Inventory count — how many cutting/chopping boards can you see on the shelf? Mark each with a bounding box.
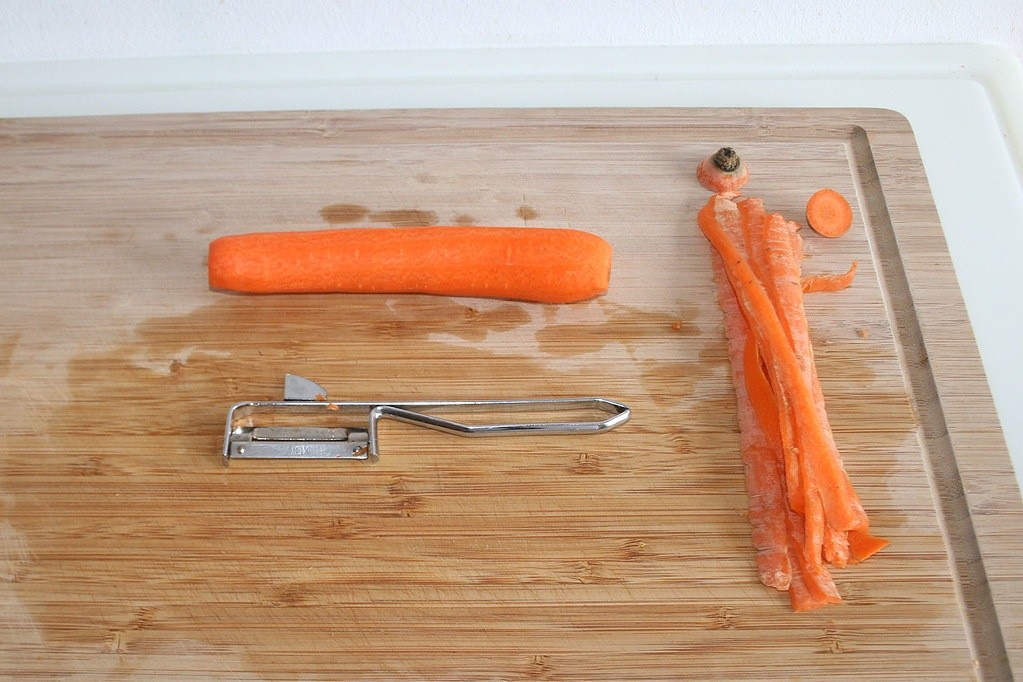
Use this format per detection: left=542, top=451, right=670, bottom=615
left=3, top=107, right=1023, bottom=681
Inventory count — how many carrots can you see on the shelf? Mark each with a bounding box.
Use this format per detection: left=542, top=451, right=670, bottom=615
left=209, top=225, right=611, bottom=303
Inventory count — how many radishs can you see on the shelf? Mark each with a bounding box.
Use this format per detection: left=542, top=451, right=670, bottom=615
left=804, top=188, right=852, bottom=237
